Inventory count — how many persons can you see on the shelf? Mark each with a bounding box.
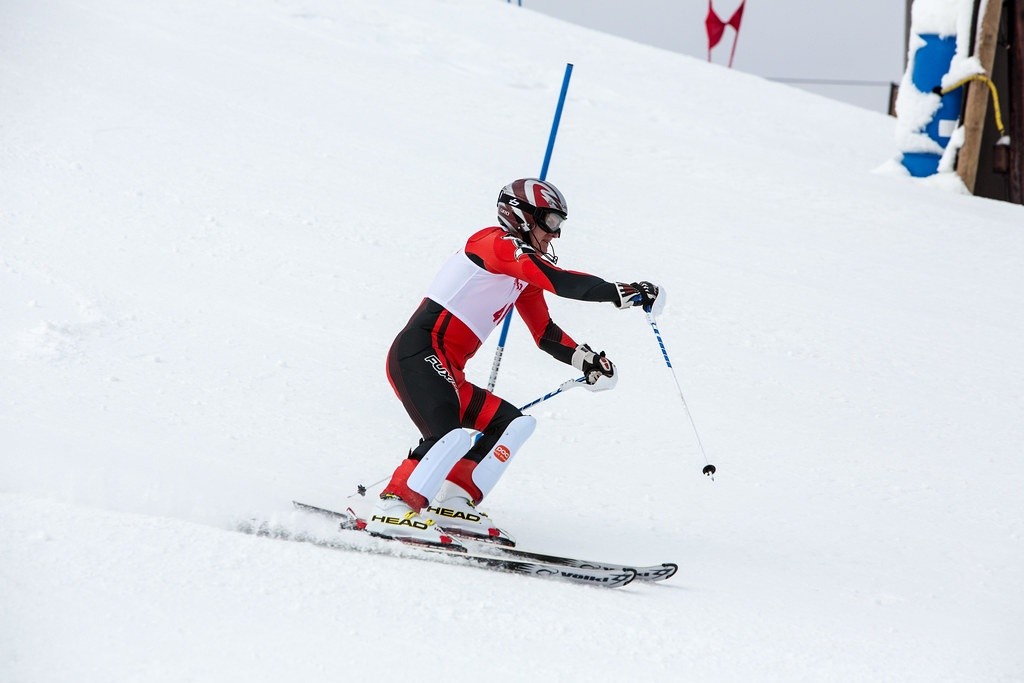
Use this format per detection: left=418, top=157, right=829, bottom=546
left=365, top=176, right=668, bottom=550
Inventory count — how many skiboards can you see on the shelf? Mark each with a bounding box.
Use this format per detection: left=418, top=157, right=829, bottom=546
left=240, top=499, right=679, bottom=589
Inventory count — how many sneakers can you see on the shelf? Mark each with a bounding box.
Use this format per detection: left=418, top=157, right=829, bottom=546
left=423, top=496, right=516, bottom=547
left=364, top=497, right=463, bottom=547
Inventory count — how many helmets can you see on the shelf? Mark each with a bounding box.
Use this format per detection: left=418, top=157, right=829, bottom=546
left=496, top=178, right=568, bottom=233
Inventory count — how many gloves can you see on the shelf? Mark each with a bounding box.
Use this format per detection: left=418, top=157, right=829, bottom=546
left=613, top=280, right=658, bottom=313
left=572, top=341, right=614, bottom=385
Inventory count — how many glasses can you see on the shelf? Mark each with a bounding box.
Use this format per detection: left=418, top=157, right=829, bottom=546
left=500, top=194, right=567, bottom=238
